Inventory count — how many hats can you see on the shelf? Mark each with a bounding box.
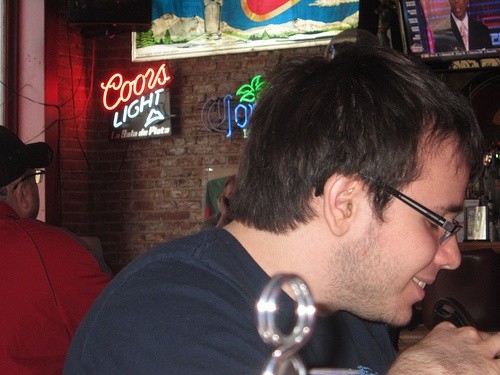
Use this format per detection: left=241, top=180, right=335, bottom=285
left=0, top=125, right=54, bottom=188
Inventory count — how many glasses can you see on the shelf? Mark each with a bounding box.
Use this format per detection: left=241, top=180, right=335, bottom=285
left=14, top=171, right=45, bottom=190
left=314, top=168, right=462, bottom=246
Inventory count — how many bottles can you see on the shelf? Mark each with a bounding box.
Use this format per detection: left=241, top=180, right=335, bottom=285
left=449, top=150, right=500, bottom=244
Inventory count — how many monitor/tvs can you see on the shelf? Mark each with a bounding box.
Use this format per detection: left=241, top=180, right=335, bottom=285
left=396, top=0, right=500, bottom=71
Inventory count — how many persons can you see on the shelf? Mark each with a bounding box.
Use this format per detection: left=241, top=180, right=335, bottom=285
left=432, top=0, right=493, bottom=53
left=61, top=28, right=500, bottom=375
left=0, top=126, right=120, bottom=375
left=198, top=175, right=235, bottom=232
